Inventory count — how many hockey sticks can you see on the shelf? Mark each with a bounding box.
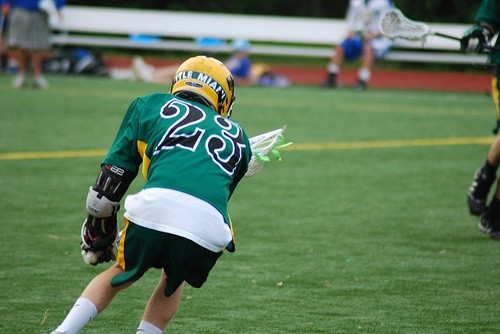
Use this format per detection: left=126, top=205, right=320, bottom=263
left=382, top=9, right=500, bottom=51
left=115, top=125, right=293, bottom=244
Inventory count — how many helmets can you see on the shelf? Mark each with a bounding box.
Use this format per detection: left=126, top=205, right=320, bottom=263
left=171, top=56, right=235, bottom=119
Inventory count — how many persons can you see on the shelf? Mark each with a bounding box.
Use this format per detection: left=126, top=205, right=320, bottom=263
left=0, top=0, right=65, bottom=89
left=49, top=55, right=253, bottom=334
left=131, top=38, right=255, bottom=86
left=460, top=0, right=500, bottom=239
left=321, top=0, right=398, bottom=90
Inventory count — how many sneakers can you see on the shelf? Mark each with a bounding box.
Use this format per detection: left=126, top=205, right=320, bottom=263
left=479, top=179, right=500, bottom=231
left=316, top=73, right=337, bottom=87
left=9, top=72, right=27, bottom=90
left=345, top=79, right=366, bottom=91
left=30, top=75, right=50, bottom=92
left=468, top=166, right=497, bottom=217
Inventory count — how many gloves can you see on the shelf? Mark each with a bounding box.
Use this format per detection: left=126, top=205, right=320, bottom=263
left=81, top=220, right=120, bottom=266
left=460, top=25, right=495, bottom=55
